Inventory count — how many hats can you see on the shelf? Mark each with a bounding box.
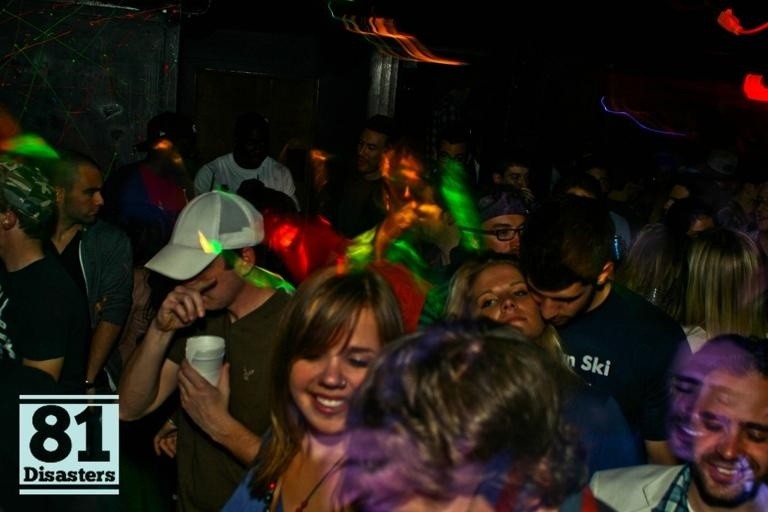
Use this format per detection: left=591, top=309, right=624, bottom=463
left=136, top=110, right=198, bottom=154
left=706, top=150, right=738, bottom=174
left=1, top=158, right=55, bottom=224
left=144, top=190, right=264, bottom=279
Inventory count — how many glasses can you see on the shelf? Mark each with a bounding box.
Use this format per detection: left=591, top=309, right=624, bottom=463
left=482, top=228, right=526, bottom=241
left=600, top=178, right=609, bottom=185
left=753, top=199, right=768, bottom=206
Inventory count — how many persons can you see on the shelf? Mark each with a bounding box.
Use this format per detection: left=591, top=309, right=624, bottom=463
left=220, top=269, right=404, bottom=512
left=446, top=257, right=641, bottom=499
left=345, top=113, right=768, bottom=339
left=333, top=321, right=586, bottom=511
left=1, top=350, right=74, bottom=512
left=52, top=155, right=133, bottom=394
left=558, top=347, right=768, bottom=512
left=116, top=190, right=298, bottom=512
left=0, top=154, right=81, bottom=387
left=518, top=194, right=683, bottom=464
left=127, top=113, right=330, bottom=187
